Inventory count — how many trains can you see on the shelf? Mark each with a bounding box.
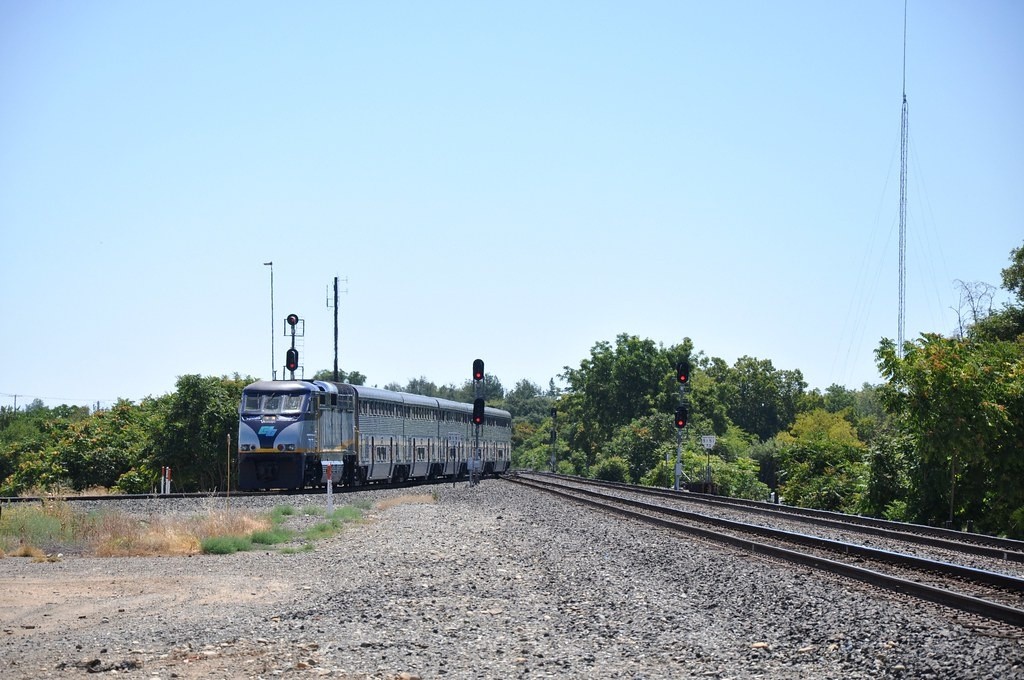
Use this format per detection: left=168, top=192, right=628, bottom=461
left=236, top=378, right=512, bottom=493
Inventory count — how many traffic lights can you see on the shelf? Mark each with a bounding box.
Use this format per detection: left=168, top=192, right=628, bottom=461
left=286, top=348, right=299, bottom=371
left=472, top=398, right=485, bottom=425
left=675, top=406, right=687, bottom=429
left=472, top=359, right=484, bottom=380
left=287, top=314, right=299, bottom=325
left=676, top=362, right=690, bottom=384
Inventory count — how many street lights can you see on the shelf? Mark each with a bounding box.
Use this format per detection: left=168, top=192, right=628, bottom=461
left=264, top=261, right=275, bottom=380
